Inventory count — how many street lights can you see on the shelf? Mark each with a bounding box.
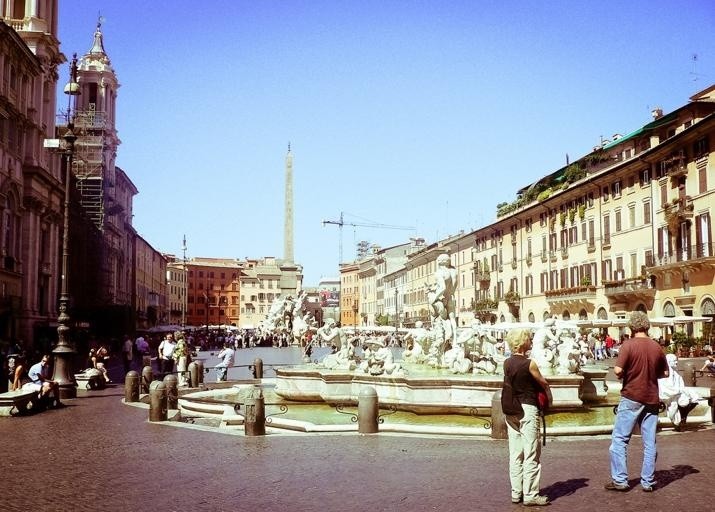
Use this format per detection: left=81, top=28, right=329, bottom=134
left=181, top=235, right=187, bottom=338
left=46, top=52, right=80, bottom=400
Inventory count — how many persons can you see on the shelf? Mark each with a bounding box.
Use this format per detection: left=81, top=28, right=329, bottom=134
left=699, top=353, right=715, bottom=376
left=654, top=336, right=671, bottom=347
left=658, top=353, right=690, bottom=427
left=578, top=334, right=630, bottom=366
left=503, top=328, right=553, bottom=506
left=318, top=254, right=583, bottom=375
left=605, top=311, right=669, bottom=491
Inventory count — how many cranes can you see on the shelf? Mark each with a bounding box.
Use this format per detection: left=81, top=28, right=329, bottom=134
left=322, top=212, right=416, bottom=280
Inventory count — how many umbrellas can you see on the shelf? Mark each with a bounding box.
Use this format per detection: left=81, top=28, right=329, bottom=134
left=577, top=316, right=713, bottom=337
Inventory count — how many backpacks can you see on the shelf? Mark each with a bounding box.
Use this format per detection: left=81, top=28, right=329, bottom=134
left=501, top=358, right=530, bottom=416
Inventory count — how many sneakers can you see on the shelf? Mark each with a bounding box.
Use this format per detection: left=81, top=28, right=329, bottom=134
left=604, top=480, right=628, bottom=491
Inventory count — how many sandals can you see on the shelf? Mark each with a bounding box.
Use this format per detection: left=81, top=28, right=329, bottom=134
left=510, top=492, right=550, bottom=506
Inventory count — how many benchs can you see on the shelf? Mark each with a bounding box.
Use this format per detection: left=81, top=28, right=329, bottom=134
left=74, top=371, right=106, bottom=390
left=1, top=383, right=42, bottom=417
